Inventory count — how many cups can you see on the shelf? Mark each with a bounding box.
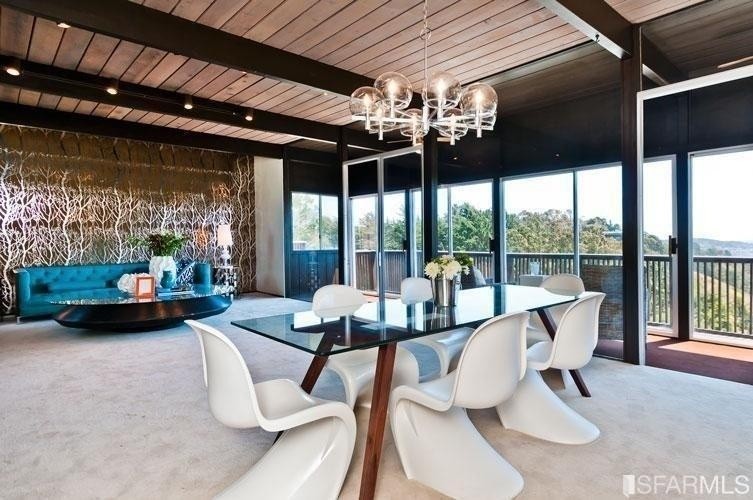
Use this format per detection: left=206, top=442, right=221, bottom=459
left=530, top=262, right=539, bottom=276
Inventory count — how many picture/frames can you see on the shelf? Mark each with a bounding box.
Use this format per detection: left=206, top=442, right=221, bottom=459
left=135, top=276, right=155, bottom=296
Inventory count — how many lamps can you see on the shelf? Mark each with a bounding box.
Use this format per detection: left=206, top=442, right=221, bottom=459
left=216, top=224, right=233, bottom=266
left=346, top=0, right=499, bottom=148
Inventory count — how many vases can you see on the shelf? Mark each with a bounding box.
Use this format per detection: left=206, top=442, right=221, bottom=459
left=116, top=272, right=149, bottom=293
left=434, top=275, right=461, bottom=307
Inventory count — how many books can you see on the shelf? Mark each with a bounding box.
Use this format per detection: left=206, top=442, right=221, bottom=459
left=157, top=286, right=193, bottom=296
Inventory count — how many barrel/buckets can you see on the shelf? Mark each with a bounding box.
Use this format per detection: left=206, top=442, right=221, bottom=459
left=193, top=263, right=211, bottom=285
left=433, top=276, right=459, bottom=307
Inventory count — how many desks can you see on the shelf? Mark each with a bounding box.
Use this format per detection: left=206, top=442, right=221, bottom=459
left=229, top=282, right=593, bottom=500
left=44, top=284, right=234, bottom=333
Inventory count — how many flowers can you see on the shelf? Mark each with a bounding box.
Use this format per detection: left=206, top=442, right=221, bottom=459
left=423, top=252, right=471, bottom=279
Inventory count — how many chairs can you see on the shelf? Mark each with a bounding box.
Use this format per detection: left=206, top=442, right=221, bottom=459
left=494, top=290, right=606, bottom=447
left=388, top=308, right=533, bottom=499
left=579, top=263, right=649, bottom=341
left=399, top=276, right=477, bottom=383
left=311, top=284, right=420, bottom=410
left=183, top=319, right=357, bottom=500
left=526, top=274, right=584, bottom=391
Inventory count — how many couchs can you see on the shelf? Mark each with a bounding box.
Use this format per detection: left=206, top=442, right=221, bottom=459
left=11, top=260, right=210, bottom=326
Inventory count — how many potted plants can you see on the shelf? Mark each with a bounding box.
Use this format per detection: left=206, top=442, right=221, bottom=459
left=126, top=229, right=190, bottom=287
